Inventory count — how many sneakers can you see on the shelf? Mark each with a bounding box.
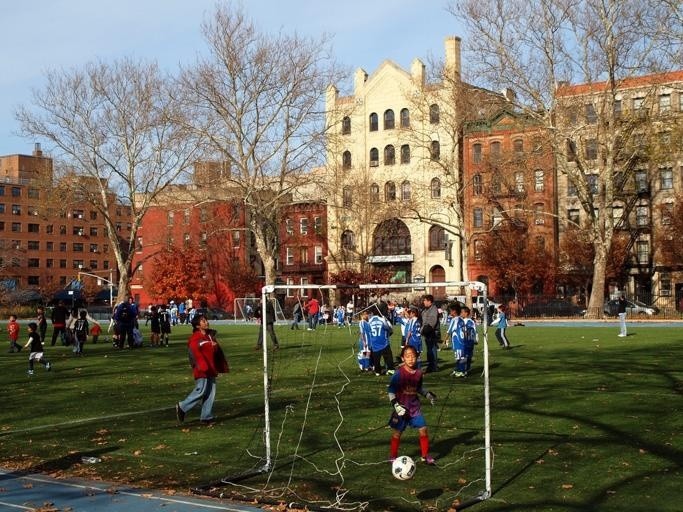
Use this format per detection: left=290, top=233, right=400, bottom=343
left=449, top=369, right=472, bottom=378
left=176, top=402, right=185, bottom=424
left=375, top=370, right=395, bottom=377
left=45, top=362, right=51, bottom=371
left=420, top=455, right=438, bottom=466
left=358, top=363, right=372, bottom=372
left=199, top=419, right=217, bottom=426
left=8, top=346, right=22, bottom=353
left=26, top=369, right=34, bottom=375
left=291, top=327, right=315, bottom=331
left=256, top=345, right=279, bottom=352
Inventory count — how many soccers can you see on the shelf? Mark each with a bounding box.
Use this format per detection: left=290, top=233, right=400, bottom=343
left=392, top=455, right=417, bottom=481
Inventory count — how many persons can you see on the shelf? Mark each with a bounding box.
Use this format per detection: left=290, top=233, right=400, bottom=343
left=356, top=293, right=511, bottom=380
left=252, top=296, right=280, bottom=351
left=242, top=291, right=356, bottom=333
left=174, top=314, right=231, bottom=427
left=387, top=346, right=438, bottom=468
left=20, top=322, right=52, bottom=376
left=5, top=295, right=197, bottom=357
left=614, top=293, right=631, bottom=341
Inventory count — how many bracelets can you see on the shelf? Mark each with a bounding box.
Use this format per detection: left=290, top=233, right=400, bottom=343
left=390, top=398, right=397, bottom=408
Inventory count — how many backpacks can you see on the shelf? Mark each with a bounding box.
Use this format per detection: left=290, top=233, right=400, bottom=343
left=119, top=303, right=131, bottom=322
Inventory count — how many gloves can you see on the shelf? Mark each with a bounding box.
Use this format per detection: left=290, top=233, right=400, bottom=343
left=394, top=401, right=409, bottom=417
left=424, top=391, right=438, bottom=406
